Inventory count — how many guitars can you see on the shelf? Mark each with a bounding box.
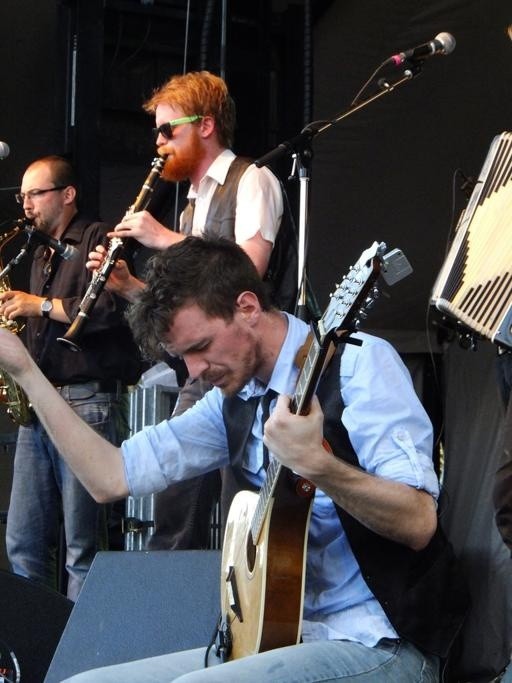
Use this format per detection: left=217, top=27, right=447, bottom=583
left=217, top=242, right=413, bottom=664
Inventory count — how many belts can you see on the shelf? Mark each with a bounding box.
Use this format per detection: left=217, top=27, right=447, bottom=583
left=56, top=379, right=122, bottom=402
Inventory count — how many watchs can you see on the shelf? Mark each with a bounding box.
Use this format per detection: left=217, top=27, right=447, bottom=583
left=40, top=296, right=53, bottom=319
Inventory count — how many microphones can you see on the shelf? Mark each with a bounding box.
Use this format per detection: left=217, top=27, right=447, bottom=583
left=0, top=140, right=10, bottom=161
left=25, top=222, right=80, bottom=263
left=389, top=31, right=456, bottom=67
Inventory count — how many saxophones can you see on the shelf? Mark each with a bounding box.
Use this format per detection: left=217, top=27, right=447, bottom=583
left=0, top=217, right=38, bottom=426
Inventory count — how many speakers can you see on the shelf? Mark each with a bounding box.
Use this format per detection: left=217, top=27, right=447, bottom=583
left=43, top=549, right=222, bottom=683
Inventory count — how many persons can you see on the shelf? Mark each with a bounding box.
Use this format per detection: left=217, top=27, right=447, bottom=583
left=85, top=69, right=295, bottom=554
left=0, top=234, right=472, bottom=683
left=1, top=155, right=132, bottom=605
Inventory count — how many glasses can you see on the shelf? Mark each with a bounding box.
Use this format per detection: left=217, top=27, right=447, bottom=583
left=150, top=114, right=217, bottom=141
left=15, top=185, right=67, bottom=204
